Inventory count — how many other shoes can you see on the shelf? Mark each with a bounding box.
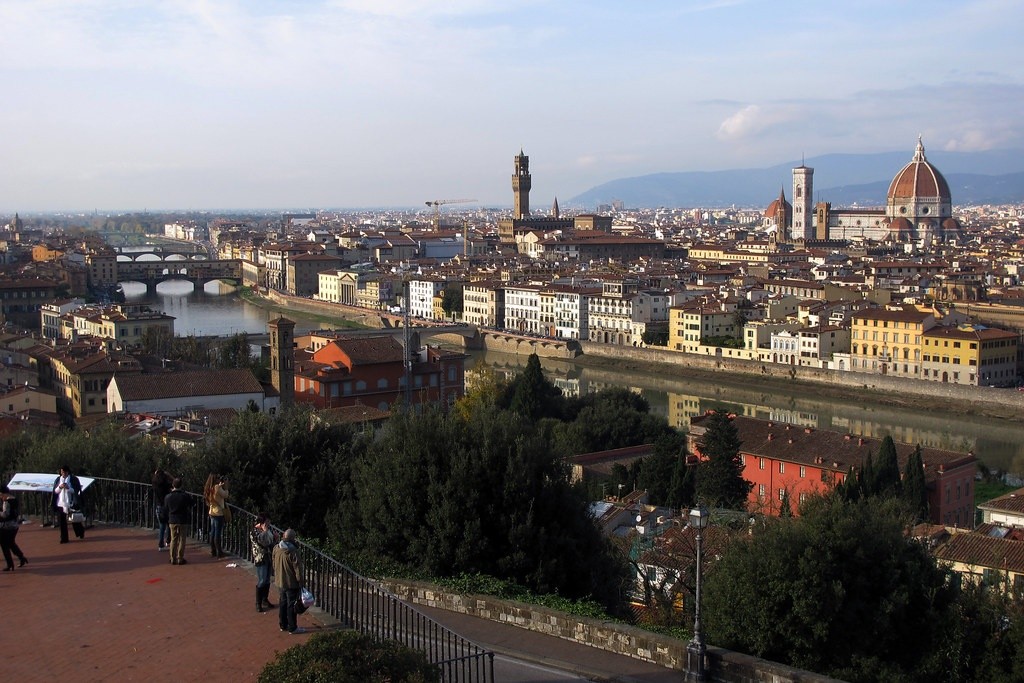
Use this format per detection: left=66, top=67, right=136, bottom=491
left=289, top=627, right=305, bottom=634
left=170, top=561, right=177, bottom=565
left=280, top=625, right=290, bottom=632
left=80, top=530, right=85, bottom=539
left=178, top=560, right=188, bottom=565
left=58, top=539, right=70, bottom=544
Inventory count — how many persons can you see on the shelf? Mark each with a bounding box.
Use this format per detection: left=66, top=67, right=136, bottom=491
left=203, top=472, right=232, bottom=558
left=0, top=486, right=28, bottom=571
left=53, top=465, right=85, bottom=544
left=164, top=478, right=195, bottom=565
left=272, top=529, right=307, bottom=634
left=152, top=468, right=172, bottom=551
left=250, top=513, right=280, bottom=612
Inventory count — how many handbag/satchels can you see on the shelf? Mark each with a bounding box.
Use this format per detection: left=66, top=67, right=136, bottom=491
left=267, top=542, right=277, bottom=565
left=223, top=507, right=231, bottom=523
left=293, top=587, right=314, bottom=614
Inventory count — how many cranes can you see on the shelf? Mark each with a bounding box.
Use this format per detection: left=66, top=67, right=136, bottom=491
left=425, top=199, right=478, bottom=233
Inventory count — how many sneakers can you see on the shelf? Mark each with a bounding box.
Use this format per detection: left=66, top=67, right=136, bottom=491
left=165, top=543, right=170, bottom=547
left=159, top=547, right=167, bottom=551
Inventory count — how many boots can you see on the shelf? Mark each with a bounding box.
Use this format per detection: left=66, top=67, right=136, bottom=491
left=210, top=539, right=217, bottom=558
left=256, top=585, right=266, bottom=613
left=262, top=584, right=274, bottom=608
left=214, top=539, right=226, bottom=557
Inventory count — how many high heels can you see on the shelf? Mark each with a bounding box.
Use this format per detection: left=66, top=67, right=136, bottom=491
left=2, top=564, right=15, bottom=572
left=17, top=557, right=29, bottom=568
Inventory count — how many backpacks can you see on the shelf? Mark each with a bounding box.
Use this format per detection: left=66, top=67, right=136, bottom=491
left=66, top=490, right=86, bottom=523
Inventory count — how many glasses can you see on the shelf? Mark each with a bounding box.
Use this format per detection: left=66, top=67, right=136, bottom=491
left=217, top=477, right=221, bottom=480
